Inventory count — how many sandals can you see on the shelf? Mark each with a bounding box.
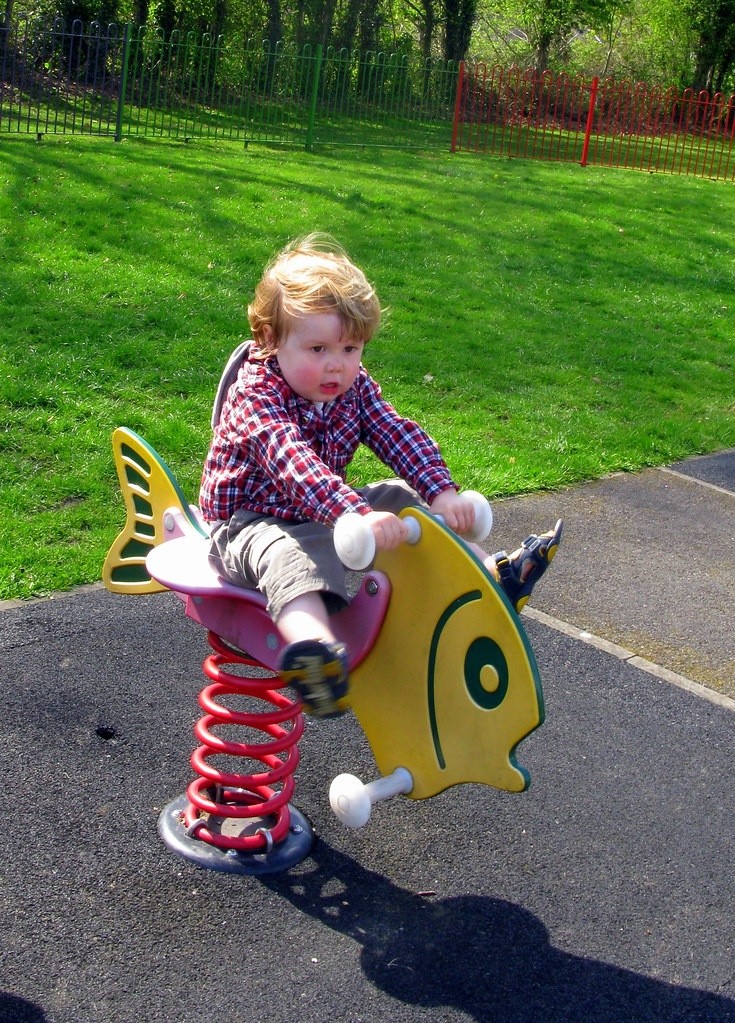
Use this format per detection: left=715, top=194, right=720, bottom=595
left=491, top=517, right=563, bottom=616
left=281, top=639, right=351, bottom=720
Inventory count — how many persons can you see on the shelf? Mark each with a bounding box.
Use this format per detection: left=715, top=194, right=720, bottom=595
left=195, top=230, right=565, bottom=721
